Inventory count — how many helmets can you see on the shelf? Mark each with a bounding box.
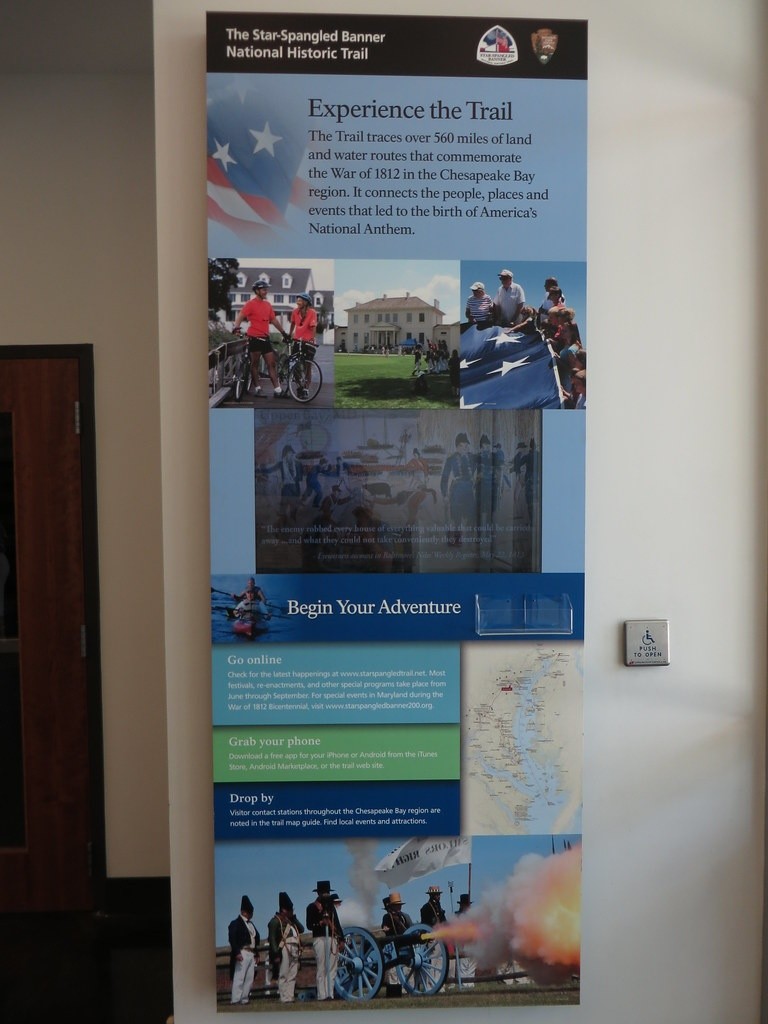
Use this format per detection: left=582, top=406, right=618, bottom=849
left=296, top=293, right=313, bottom=305
left=252, top=280, right=271, bottom=289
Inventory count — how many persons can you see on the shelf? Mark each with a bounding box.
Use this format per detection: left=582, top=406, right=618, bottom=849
left=232, top=577, right=271, bottom=623
left=465, top=281, right=493, bottom=323
left=232, top=280, right=318, bottom=399
left=381, top=337, right=423, bottom=376
left=380, top=886, right=535, bottom=998
left=424, top=338, right=460, bottom=398
left=493, top=269, right=586, bottom=409
left=227, top=880, right=346, bottom=1005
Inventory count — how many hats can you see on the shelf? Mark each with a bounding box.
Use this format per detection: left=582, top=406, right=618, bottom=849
left=381, top=897, right=391, bottom=909
left=240, top=895, right=254, bottom=911
left=470, top=282, right=484, bottom=291
left=425, top=886, right=443, bottom=894
left=457, top=894, right=472, bottom=904
left=279, top=892, right=293, bottom=907
left=389, top=894, right=406, bottom=905
left=245, top=586, right=255, bottom=593
left=497, top=269, right=513, bottom=278
left=330, top=894, right=342, bottom=903
left=312, top=881, right=335, bottom=892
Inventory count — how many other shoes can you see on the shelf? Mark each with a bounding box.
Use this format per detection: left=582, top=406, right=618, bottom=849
left=275, top=389, right=289, bottom=399
left=255, top=388, right=266, bottom=398
left=303, top=389, right=309, bottom=399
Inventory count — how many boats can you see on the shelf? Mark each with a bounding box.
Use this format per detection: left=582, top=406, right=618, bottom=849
left=233, top=619, right=270, bottom=639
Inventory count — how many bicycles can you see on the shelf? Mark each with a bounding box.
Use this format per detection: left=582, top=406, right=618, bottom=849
left=233, top=337, right=322, bottom=404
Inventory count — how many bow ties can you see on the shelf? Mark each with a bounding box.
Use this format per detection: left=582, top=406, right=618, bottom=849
left=245, top=920, right=250, bottom=923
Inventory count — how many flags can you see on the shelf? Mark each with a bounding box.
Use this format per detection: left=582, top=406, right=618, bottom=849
left=374, top=835, right=471, bottom=890
